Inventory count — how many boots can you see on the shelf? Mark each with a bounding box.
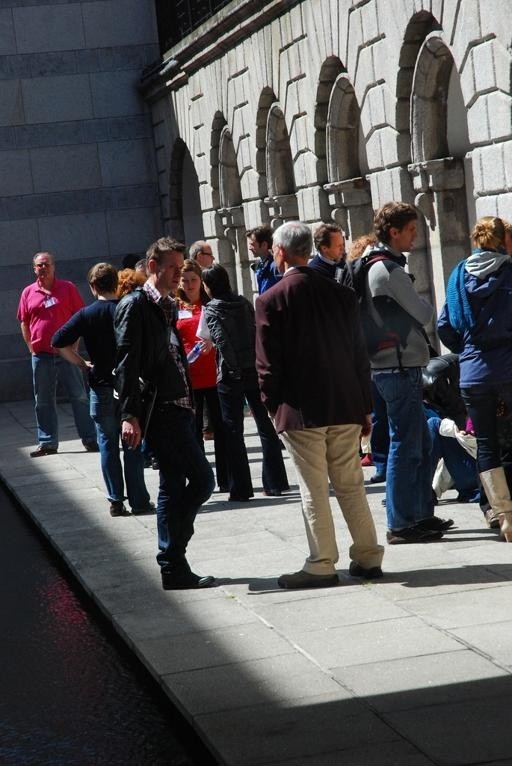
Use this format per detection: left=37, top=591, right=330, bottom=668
left=482, top=462, right=512, bottom=545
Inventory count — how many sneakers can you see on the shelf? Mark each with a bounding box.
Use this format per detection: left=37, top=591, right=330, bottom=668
left=85, top=442, right=99, bottom=453
left=130, top=498, right=156, bottom=515
left=30, top=444, right=47, bottom=457
left=108, top=501, right=127, bottom=518
left=347, top=561, right=384, bottom=580
left=276, top=571, right=340, bottom=588
left=216, top=481, right=285, bottom=502
left=161, top=568, right=215, bottom=592
left=385, top=513, right=456, bottom=545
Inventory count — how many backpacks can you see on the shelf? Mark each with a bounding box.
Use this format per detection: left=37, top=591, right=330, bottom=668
left=336, top=250, right=405, bottom=356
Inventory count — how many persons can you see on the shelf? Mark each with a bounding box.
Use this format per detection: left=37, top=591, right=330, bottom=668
left=16, top=200, right=511, bottom=590
